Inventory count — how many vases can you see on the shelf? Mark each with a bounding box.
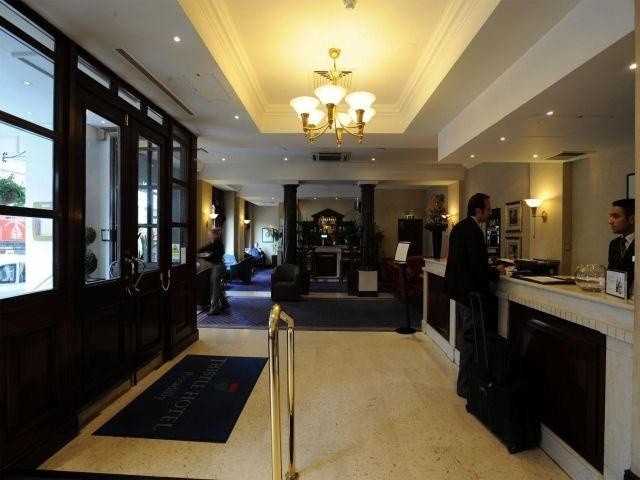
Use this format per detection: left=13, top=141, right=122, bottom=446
left=427, top=193, right=448, bottom=224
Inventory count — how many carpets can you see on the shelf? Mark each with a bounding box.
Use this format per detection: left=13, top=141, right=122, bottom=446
left=620, top=238, right=626, bottom=256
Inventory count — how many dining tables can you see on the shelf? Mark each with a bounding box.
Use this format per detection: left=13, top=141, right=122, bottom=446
left=296, top=219, right=313, bottom=295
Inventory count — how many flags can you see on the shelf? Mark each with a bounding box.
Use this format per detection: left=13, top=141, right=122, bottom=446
left=424, top=224, right=448, bottom=259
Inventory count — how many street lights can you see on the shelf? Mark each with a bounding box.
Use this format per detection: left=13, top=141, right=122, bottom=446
left=478, top=330, right=520, bottom=386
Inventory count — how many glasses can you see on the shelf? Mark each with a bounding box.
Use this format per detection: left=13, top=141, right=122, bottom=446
left=290, top=48, right=376, bottom=148
left=523, top=198, right=547, bottom=223
left=206, top=213, right=219, bottom=226
left=242, top=220, right=251, bottom=224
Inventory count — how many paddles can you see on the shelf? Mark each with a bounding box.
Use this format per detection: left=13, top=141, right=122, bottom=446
left=91, top=354, right=268, bottom=443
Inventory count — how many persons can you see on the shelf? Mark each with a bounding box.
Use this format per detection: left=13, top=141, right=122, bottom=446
left=441, top=191, right=508, bottom=399
left=197, top=227, right=231, bottom=316
left=603, top=196, right=636, bottom=299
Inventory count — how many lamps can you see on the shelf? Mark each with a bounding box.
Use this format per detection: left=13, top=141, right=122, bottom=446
left=271, top=264, right=301, bottom=301
left=230, top=257, right=255, bottom=284
left=223, top=253, right=238, bottom=284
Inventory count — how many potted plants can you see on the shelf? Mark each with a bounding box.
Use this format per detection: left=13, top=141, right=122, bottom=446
left=262, top=227, right=274, bottom=244
left=605, top=269, right=628, bottom=301
left=505, top=235, right=522, bottom=259
left=505, top=200, right=522, bottom=233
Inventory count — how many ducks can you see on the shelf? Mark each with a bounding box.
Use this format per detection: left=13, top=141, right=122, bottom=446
left=466, top=377, right=542, bottom=454
